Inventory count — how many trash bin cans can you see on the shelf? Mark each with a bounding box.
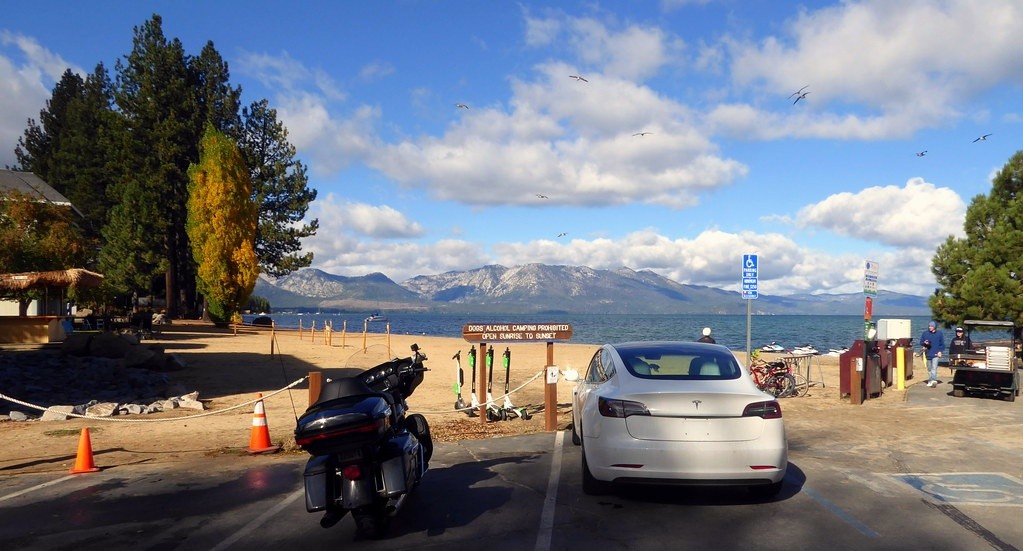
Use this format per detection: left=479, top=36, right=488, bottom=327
left=840, top=339, right=881, bottom=398
left=876, top=338, right=893, bottom=388
left=890, top=337, right=914, bottom=380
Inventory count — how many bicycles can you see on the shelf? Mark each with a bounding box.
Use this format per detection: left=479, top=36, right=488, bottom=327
left=744, top=347, right=811, bottom=400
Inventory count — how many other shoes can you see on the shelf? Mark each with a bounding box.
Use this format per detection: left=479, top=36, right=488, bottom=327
left=926, top=381, right=937, bottom=388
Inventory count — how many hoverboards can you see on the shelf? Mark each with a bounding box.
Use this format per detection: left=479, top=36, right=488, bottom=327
left=485, top=345, right=507, bottom=415
left=500, top=344, right=530, bottom=421
left=450, top=350, right=473, bottom=417
left=467, top=346, right=493, bottom=421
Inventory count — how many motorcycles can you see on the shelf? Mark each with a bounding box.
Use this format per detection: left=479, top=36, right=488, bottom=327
left=291, top=345, right=436, bottom=538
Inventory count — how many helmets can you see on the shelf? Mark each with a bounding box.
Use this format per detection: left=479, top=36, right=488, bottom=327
left=703, top=327, right=711, bottom=336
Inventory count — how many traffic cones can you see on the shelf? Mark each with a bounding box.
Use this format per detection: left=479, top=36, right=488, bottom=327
left=244, top=391, right=283, bottom=455
left=67, top=426, right=102, bottom=476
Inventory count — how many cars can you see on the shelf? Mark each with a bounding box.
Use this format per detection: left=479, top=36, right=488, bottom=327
left=948, top=319, right=1021, bottom=402
left=562, top=338, right=791, bottom=498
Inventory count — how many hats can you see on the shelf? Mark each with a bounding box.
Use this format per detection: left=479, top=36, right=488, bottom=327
left=928, top=322, right=935, bottom=328
left=954, top=328, right=964, bottom=332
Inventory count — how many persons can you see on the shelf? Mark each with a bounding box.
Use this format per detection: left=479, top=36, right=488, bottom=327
left=949, top=326, right=976, bottom=355
left=697, top=327, right=716, bottom=343
left=919, top=321, right=945, bottom=387
